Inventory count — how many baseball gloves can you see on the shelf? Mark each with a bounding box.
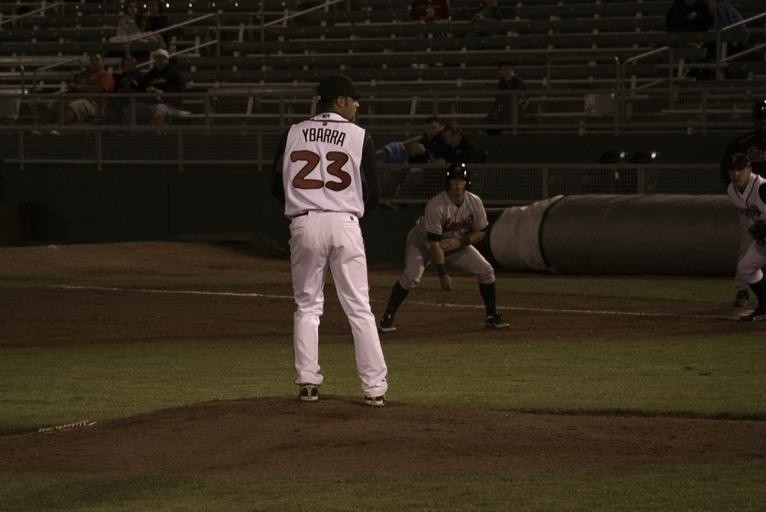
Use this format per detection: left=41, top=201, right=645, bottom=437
left=748, top=220, right=765, bottom=246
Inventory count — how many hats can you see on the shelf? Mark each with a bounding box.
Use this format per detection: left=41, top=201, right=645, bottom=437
left=316, top=73, right=370, bottom=101
left=727, top=153, right=751, bottom=171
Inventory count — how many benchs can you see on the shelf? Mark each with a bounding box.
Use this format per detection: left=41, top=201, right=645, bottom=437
left=0, top=0, right=764, bottom=168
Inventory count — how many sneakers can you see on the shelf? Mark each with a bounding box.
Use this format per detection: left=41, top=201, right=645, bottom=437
left=740, top=308, right=766, bottom=323
left=485, top=313, right=510, bottom=330
left=378, top=318, right=396, bottom=333
left=299, top=383, right=319, bottom=403
left=734, top=290, right=751, bottom=306
left=364, top=393, right=386, bottom=407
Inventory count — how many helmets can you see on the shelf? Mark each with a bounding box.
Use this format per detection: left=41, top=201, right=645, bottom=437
left=445, top=162, right=472, bottom=193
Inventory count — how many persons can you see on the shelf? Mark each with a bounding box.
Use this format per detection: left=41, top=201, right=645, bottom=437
left=410, top=0, right=457, bottom=43
left=62, top=1, right=185, bottom=125
left=410, top=115, right=464, bottom=165
left=378, top=159, right=510, bottom=335
left=484, top=53, right=533, bottom=126
left=723, top=148, right=766, bottom=326
left=279, top=74, right=391, bottom=409
left=470, top=0, right=503, bottom=41
